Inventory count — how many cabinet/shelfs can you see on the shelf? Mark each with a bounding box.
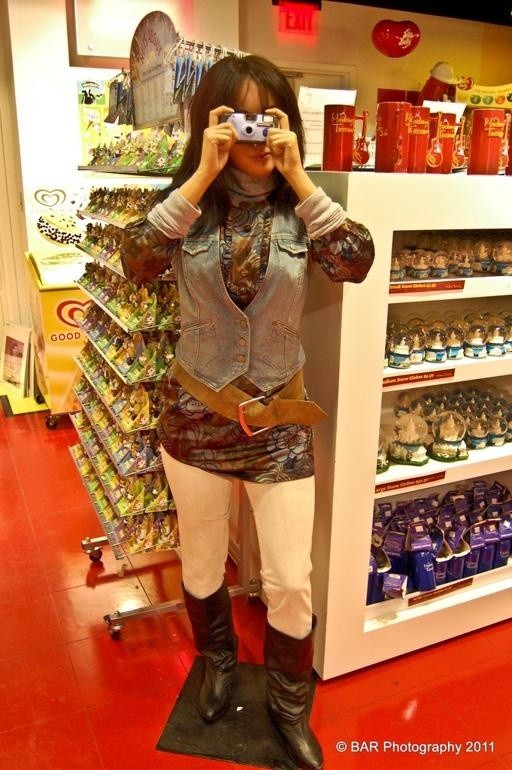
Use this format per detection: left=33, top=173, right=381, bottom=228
left=68, top=165, right=180, bottom=560
left=296, top=170, right=512, bottom=681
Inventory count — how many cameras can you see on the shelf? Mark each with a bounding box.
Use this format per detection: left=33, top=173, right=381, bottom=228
left=219, top=111, right=275, bottom=144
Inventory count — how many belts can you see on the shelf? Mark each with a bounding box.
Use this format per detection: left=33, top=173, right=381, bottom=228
left=170, top=356, right=329, bottom=443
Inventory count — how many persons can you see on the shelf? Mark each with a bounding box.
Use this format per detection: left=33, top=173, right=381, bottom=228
left=117, top=52, right=376, bottom=770
left=376, top=238, right=512, bottom=479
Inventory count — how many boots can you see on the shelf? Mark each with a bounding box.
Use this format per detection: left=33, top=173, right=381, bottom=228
left=179, top=573, right=241, bottom=725
left=261, top=613, right=325, bottom=769
